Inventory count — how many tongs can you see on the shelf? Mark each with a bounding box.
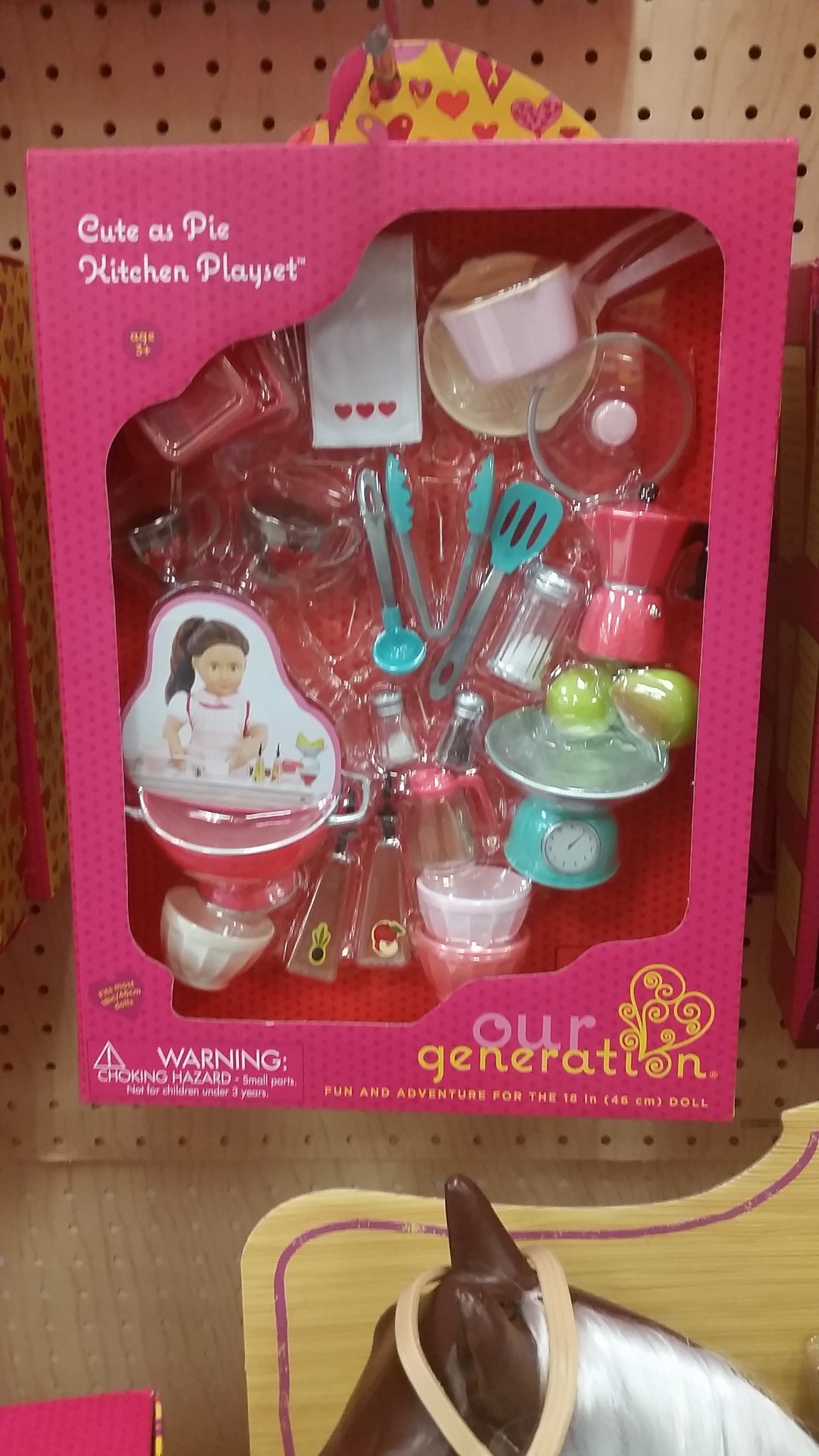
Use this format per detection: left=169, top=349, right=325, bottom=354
left=383, top=451, right=495, bottom=635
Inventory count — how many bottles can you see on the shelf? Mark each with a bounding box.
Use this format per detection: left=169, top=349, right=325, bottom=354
left=479, top=554, right=592, bottom=692
left=277, top=682, right=504, bottom=979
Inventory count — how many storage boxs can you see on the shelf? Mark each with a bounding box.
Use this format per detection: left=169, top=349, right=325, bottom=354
left=17, top=138, right=801, bottom=1131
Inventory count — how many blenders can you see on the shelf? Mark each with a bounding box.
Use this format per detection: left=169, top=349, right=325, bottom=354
left=573, top=480, right=711, bottom=663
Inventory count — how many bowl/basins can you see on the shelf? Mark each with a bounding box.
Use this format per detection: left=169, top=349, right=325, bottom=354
left=161, top=882, right=277, bottom=991
left=410, top=868, right=535, bottom=1002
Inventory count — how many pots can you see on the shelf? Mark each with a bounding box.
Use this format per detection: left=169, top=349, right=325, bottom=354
left=419, top=211, right=716, bottom=439
left=125, top=770, right=372, bottom=917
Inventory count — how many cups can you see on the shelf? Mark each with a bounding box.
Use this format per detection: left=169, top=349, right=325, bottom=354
left=112, top=485, right=222, bottom=582
left=243, top=495, right=367, bottom=593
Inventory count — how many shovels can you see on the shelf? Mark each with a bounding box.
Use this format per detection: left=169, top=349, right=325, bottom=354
left=427, top=480, right=565, bottom=703
